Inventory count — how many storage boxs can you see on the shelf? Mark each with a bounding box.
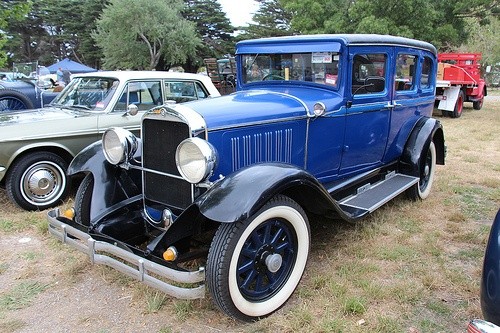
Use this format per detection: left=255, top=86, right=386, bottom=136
left=437, top=63, right=462, bottom=80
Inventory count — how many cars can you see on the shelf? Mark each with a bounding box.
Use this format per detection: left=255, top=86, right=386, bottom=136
left=47, top=32, right=447, bottom=324
left=0, top=72, right=27, bottom=80
left=0, top=80, right=139, bottom=112
left=210, top=59, right=238, bottom=84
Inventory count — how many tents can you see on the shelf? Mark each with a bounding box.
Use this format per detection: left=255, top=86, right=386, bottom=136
left=34, top=58, right=98, bottom=74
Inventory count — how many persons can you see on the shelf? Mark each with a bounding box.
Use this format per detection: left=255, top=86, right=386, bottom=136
left=56, top=66, right=63, bottom=80
left=62, top=67, right=71, bottom=86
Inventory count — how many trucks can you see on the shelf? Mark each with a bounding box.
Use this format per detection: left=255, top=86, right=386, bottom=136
left=393, top=52, right=488, bottom=118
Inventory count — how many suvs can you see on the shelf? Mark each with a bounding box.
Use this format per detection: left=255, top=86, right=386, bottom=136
left=0, top=70, right=222, bottom=212
